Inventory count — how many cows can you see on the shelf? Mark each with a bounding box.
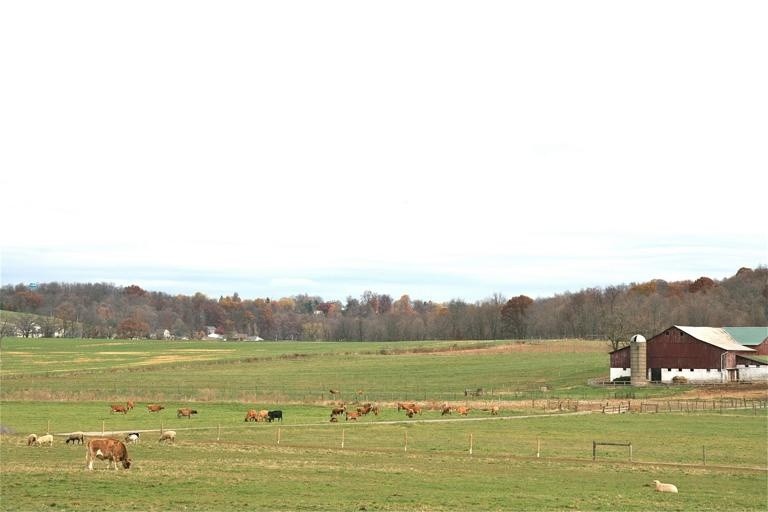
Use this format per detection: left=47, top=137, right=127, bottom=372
left=397, top=403, right=424, bottom=418
left=244, top=409, right=283, bottom=423
left=85, top=438, right=133, bottom=471
left=145, top=405, right=164, bottom=414
left=126, top=400, right=134, bottom=411
left=110, top=405, right=128, bottom=415
left=177, top=408, right=198, bottom=419
left=329, top=403, right=381, bottom=424
left=441, top=403, right=472, bottom=418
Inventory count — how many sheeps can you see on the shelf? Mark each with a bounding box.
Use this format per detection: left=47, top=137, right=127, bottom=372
left=158, top=430, right=177, bottom=444
left=125, top=433, right=140, bottom=446
left=25, top=433, right=36, bottom=446
left=65, top=431, right=84, bottom=445
left=35, top=434, right=54, bottom=448
